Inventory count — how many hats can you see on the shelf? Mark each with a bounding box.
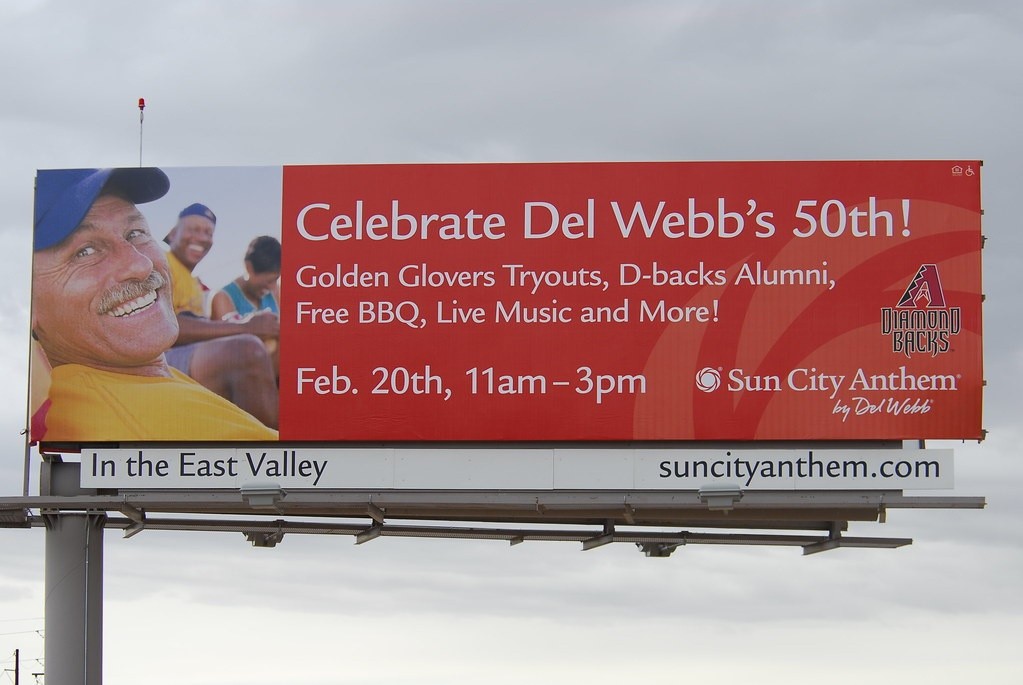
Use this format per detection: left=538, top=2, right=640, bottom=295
left=32, top=167, right=170, bottom=249
left=162, top=203, right=216, bottom=244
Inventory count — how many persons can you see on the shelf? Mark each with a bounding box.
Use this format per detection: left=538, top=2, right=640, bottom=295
left=210, top=236, right=281, bottom=322
left=32, top=168, right=279, bottom=441
left=164, top=202, right=280, bottom=431
